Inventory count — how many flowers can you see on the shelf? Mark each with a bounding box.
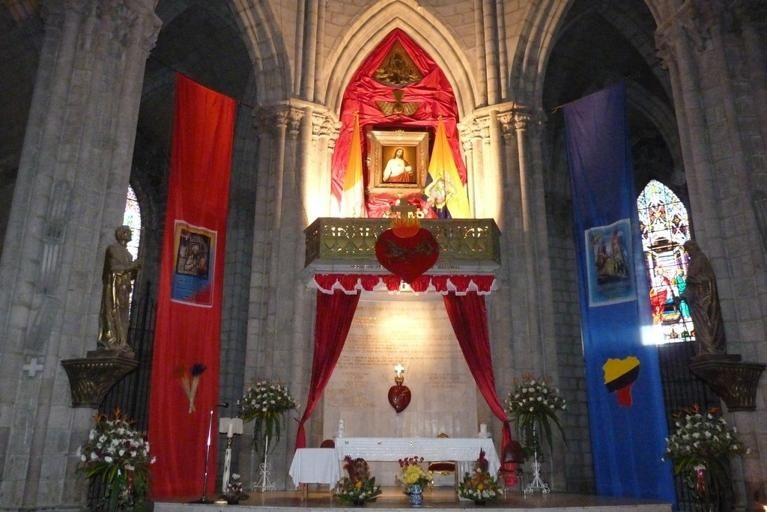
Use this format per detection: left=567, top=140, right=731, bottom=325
left=391, top=455, right=434, bottom=491
left=457, top=449, right=505, bottom=500
left=76, top=406, right=158, bottom=512
left=503, top=379, right=571, bottom=454
left=335, top=455, right=382, bottom=503
left=234, top=377, right=301, bottom=451
left=661, top=402, right=753, bottom=512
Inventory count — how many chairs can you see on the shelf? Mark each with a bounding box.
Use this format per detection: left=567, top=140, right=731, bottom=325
left=320, top=439, right=335, bottom=449
left=428, top=434, right=459, bottom=504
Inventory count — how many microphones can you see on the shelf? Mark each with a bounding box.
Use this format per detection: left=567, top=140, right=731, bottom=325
left=217, top=402, right=229, bottom=408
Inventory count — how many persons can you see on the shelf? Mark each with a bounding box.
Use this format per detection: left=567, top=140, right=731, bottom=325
left=674, top=240, right=726, bottom=354
left=383, top=148, right=412, bottom=183
left=96, top=225, right=143, bottom=350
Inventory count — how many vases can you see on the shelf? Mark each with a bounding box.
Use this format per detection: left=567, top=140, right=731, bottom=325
left=410, top=485, right=424, bottom=508
left=475, top=498, right=486, bottom=506
left=352, top=498, right=364, bottom=507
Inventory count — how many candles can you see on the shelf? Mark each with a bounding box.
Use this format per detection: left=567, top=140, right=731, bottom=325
left=480, top=423, right=487, bottom=434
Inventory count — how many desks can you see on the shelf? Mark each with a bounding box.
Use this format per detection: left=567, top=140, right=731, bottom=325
left=289, top=447, right=341, bottom=503
left=336, top=435, right=501, bottom=503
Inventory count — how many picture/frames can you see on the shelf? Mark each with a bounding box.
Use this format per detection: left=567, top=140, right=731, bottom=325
left=367, top=130, right=431, bottom=196
